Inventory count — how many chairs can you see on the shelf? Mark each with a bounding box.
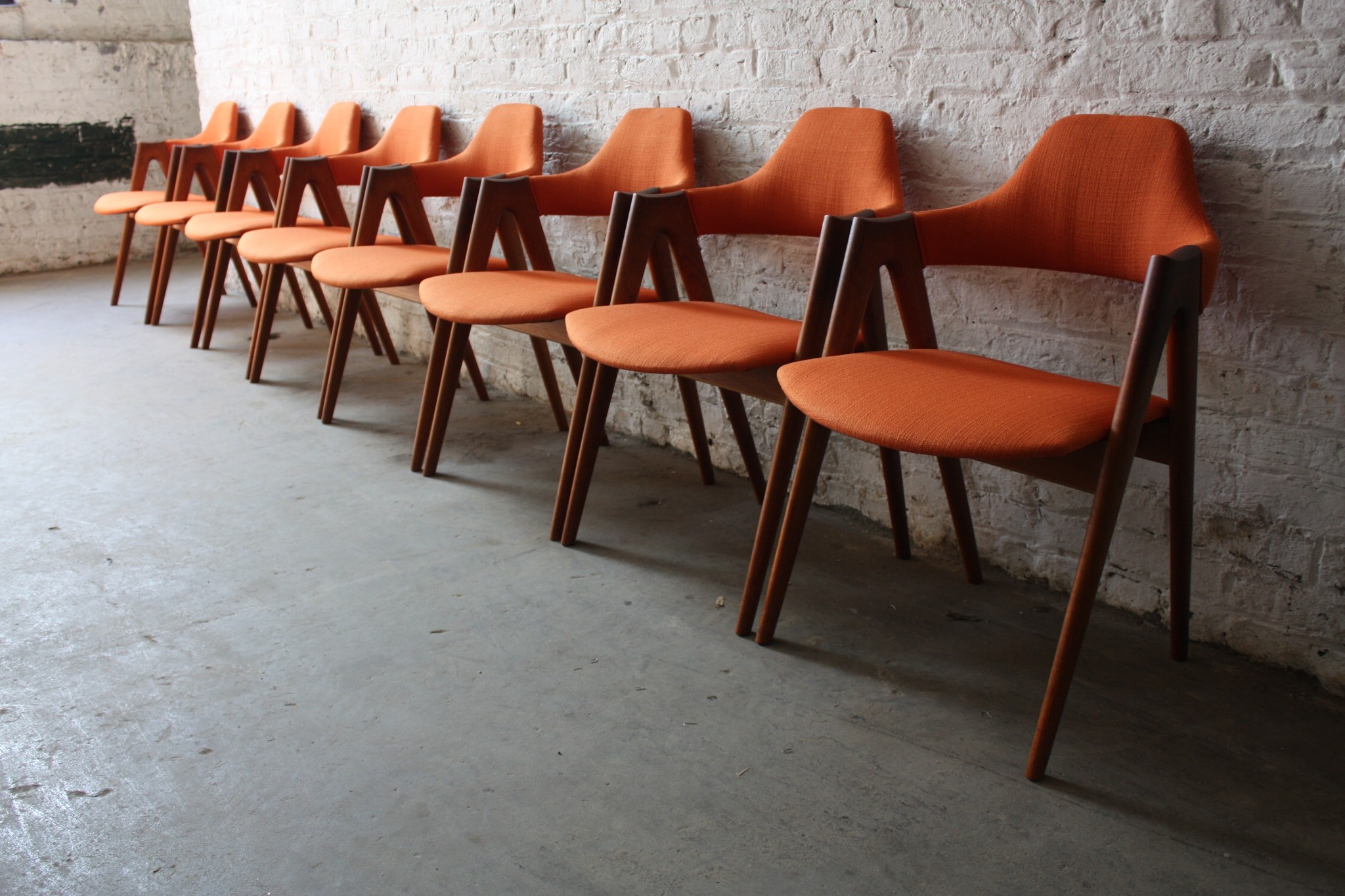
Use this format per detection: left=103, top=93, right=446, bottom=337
left=185, top=102, right=334, bottom=379
left=93, top=101, right=237, bottom=324
left=311, top=103, right=568, bottom=435
left=756, top=114, right=1217, bottom=780
left=560, top=107, right=909, bottom=633
left=135, top=101, right=312, bottom=347
left=237, top=106, right=400, bottom=419
left=420, top=106, right=713, bottom=485
